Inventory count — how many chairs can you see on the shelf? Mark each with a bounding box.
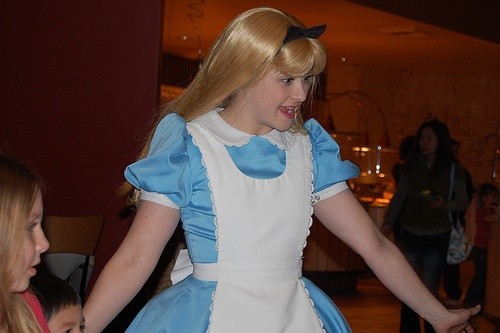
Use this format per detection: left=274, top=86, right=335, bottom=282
left=42, top=215, right=105, bottom=304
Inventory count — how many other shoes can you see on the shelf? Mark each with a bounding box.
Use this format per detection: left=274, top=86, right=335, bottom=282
left=447, top=298, right=457, bottom=306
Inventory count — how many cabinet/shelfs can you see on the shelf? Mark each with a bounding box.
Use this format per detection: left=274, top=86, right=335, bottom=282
left=304, top=207, right=395, bottom=271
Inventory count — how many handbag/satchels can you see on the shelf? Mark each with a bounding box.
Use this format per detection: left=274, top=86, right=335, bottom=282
left=446, top=224, right=472, bottom=263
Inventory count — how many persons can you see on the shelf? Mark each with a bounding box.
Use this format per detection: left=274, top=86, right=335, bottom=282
left=0, top=153, right=52, bottom=333
left=29, top=6, right=500, bottom=333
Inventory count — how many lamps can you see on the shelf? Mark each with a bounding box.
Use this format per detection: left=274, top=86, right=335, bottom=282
left=321, top=89, right=391, bottom=151
